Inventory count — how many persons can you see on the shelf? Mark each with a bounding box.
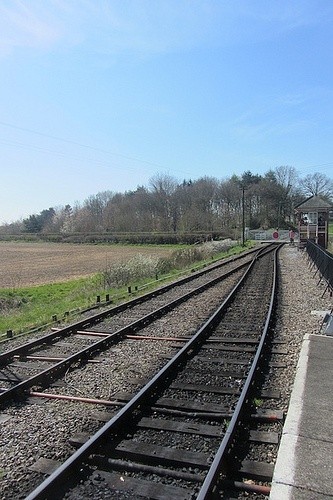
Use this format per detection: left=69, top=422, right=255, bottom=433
left=288, top=229, right=296, bottom=246
